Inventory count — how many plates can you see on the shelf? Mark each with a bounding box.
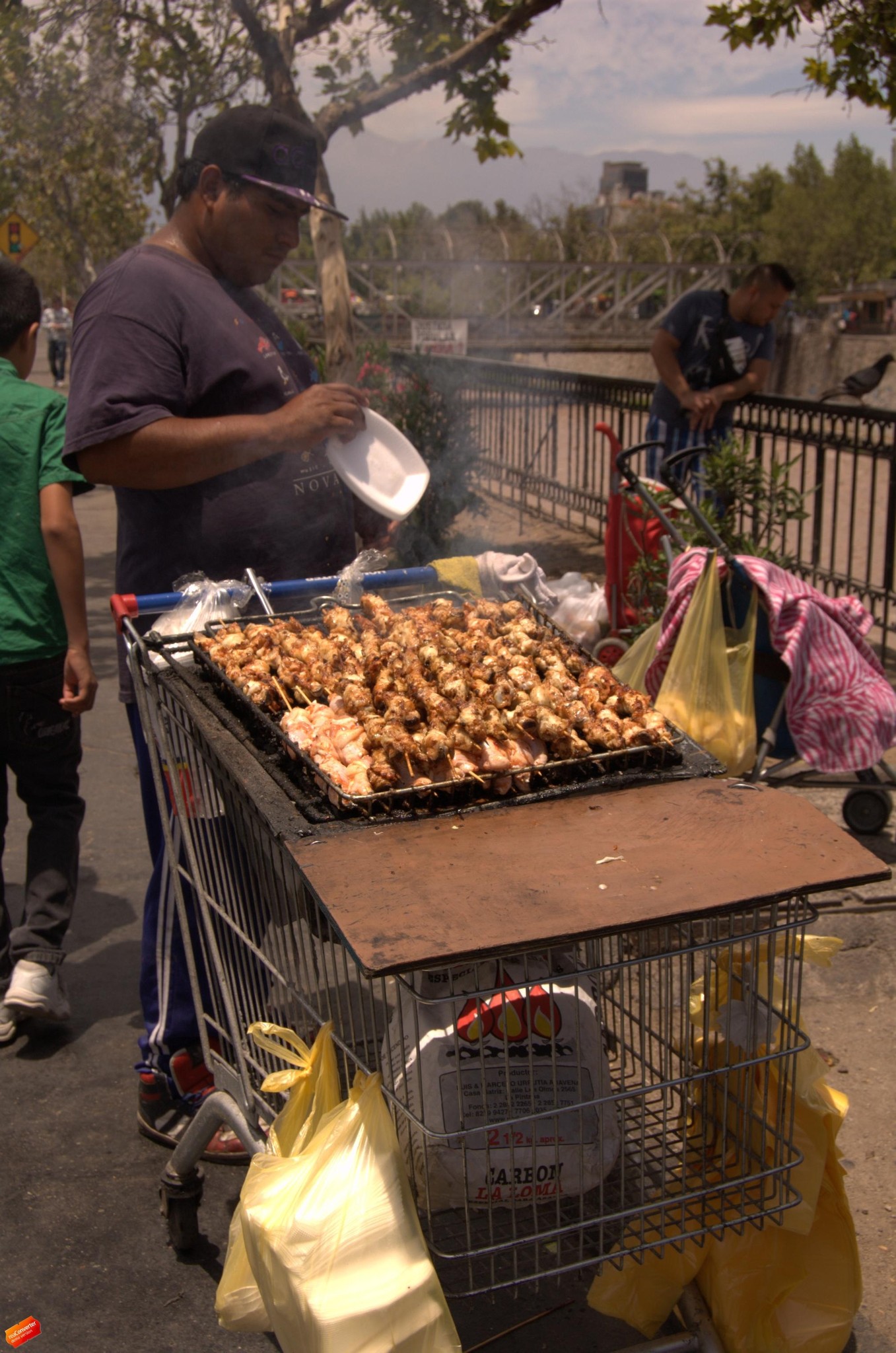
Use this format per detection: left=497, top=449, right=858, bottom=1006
left=326, top=403, right=431, bottom=522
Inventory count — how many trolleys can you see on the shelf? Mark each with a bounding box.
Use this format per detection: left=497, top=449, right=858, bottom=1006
left=106, top=553, right=823, bottom=1321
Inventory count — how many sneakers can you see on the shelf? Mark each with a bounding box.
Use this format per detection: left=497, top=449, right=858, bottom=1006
left=0, top=1000, right=17, bottom=1041
left=5, top=959, right=72, bottom=1025
left=136, top=1072, right=270, bottom=1158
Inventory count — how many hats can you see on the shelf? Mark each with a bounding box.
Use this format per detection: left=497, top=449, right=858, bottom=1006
left=193, top=105, right=351, bottom=222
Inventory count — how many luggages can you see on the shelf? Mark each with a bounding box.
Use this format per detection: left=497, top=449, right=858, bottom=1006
left=593, top=421, right=692, bottom=666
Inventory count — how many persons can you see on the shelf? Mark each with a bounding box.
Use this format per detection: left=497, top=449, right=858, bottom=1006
left=647, top=262, right=795, bottom=519
left=41, top=292, right=73, bottom=388
left=63, top=100, right=401, bottom=1161
left=0, top=259, right=99, bottom=1051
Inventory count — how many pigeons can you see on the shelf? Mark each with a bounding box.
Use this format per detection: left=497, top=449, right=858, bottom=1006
left=818, top=352, right=896, bottom=407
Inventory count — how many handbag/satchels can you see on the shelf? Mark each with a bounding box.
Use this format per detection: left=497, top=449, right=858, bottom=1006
left=381, top=951, right=621, bottom=1213
left=653, top=548, right=760, bottom=777
